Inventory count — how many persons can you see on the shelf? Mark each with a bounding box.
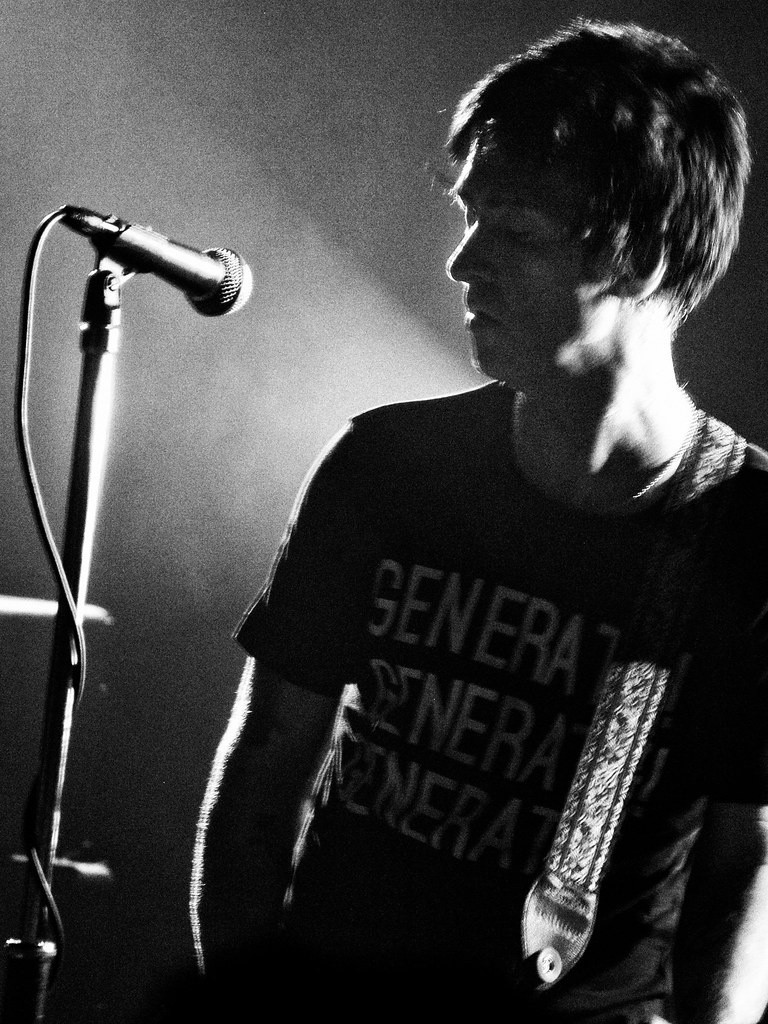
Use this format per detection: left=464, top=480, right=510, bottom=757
left=189, top=21, right=767, bottom=1024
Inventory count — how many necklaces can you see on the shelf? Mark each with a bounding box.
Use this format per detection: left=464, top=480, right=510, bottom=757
left=602, top=388, right=698, bottom=510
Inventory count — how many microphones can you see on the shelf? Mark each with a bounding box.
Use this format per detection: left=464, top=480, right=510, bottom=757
left=58, top=205, right=254, bottom=317
